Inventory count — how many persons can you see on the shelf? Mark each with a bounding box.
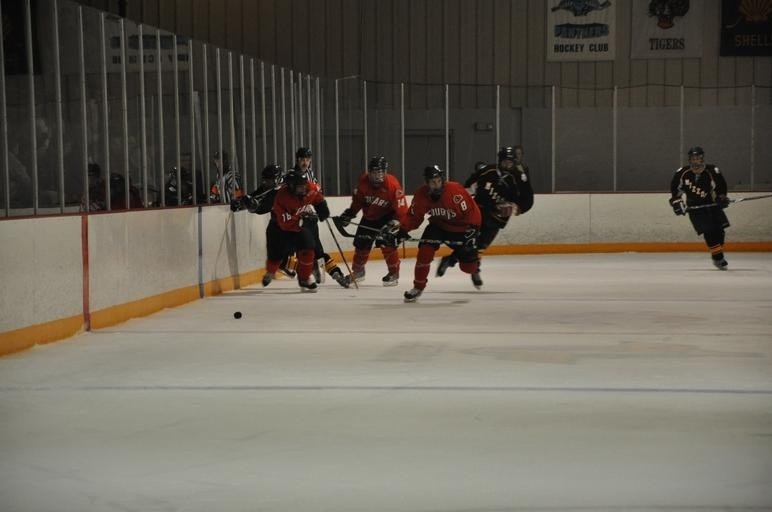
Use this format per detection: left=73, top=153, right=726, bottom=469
left=338, top=155, right=409, bottom=284
left=669, top=146, right=731, bottom=266
left=79, top=148, right=344, bottom=289
left=437, top=144, right=533, bottom=277
left=396, top=165, right=482, bottom=299
left=470, top=161, right=486, bottom=192
left=513, top=145, right=530, bottom=176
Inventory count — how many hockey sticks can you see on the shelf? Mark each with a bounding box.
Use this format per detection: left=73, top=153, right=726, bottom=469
left=328, top=222, right=361, bottom=297
left=332, top=216, right=463, bottom=246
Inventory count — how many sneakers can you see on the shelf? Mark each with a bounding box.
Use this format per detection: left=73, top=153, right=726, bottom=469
left=346, top=267, right=365, bottom=280
left=382, top=272, right=399, bottom=281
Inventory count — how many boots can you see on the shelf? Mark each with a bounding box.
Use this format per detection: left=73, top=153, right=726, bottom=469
left=298, top=280, right=316, bottom=289
left=280, top=267, right=296, bottom=277
left=404, top=288, right=423, bottom=299
left=438, top=256, right=450, bottom=277
left=713, top=259, right=728, bottom=267
left=472, top=269, right=483, bottom=285
left=332, top=273, right=349, bottom=288
left=262, top=272, right=272, bottom=286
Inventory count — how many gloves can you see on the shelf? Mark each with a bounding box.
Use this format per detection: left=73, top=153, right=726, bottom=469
left=462, top=227, right=479, bottom=255
left=302, top=215, right=318, bottom=241
left=379, top=220, right=401, bottom=238
left=496, top=202, right=519, bottom=218
left=314, top=200, right=330, bottom=222
left=386, top=232, right=410, bottom=248
left=243, top=195, right=260, bottom=213
left=715, top=195, right=729, bottom=209
left=672, top=198, right=687, bottom=216
left=230, top=199, right=245, bottom=212
left=339, top=209, right=356, bottom=226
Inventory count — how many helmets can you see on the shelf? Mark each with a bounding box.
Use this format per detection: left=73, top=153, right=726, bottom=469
left=497, top=145, right=516, bottom=173
left=688, top=146, right=705, bottom=168
left=366, top=155, right=390, bottom=186
left=260, top=146, right=313, bottom=197
left=422, top=166, right=444, bottom=196
